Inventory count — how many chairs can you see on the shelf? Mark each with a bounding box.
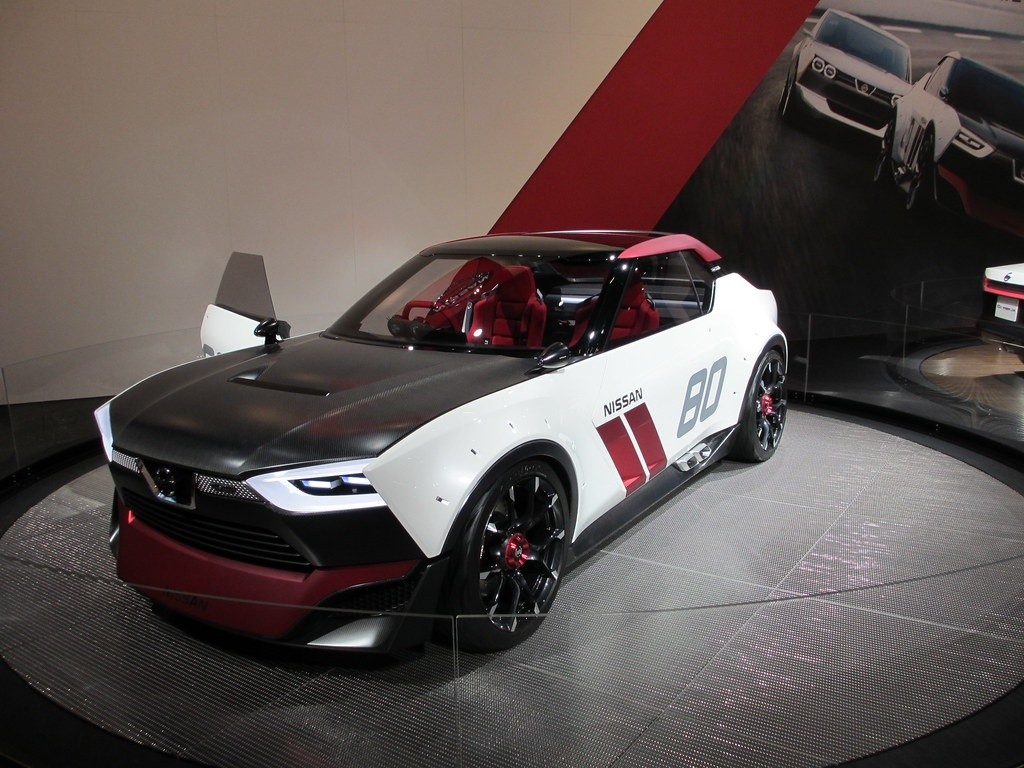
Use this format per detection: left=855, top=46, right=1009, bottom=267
left=466, top=266, right=547, bottom=348
left=567, top=267, right=660, bottom=351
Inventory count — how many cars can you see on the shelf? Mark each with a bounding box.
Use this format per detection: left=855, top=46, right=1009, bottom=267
left=91, top=231, right=792, bottom=659
left=778, top=9, right=912, bottom=142
left=979, top=261, right=1024, bottom=363
left=874, top=53, right=1024, bottom=236
